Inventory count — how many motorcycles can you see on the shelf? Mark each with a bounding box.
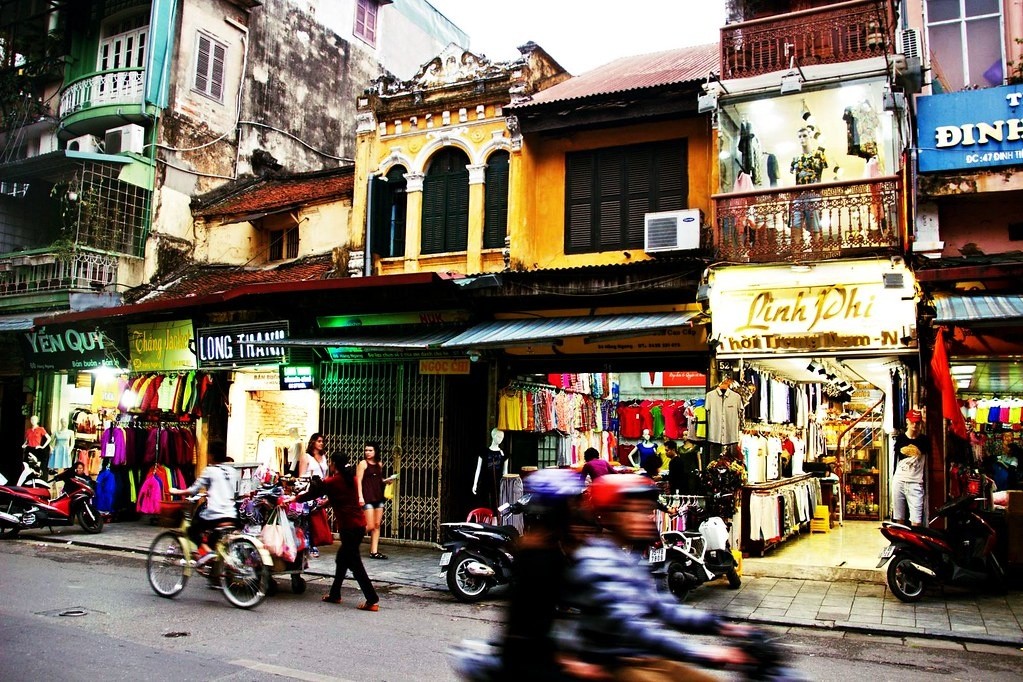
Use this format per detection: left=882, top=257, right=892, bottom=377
left=0, top=463, right=105, bottom=538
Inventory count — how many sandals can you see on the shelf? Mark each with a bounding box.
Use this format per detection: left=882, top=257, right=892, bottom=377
left=370, top=553, right=388, bottom=560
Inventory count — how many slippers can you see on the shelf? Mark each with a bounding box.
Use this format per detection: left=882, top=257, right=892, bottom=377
left=321, top=596, right=342, bottom=604
left=357, top=603, right=379, bottom=611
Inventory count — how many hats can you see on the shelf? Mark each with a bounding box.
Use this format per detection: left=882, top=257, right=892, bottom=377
left=907, top=410, right=922, bottom=421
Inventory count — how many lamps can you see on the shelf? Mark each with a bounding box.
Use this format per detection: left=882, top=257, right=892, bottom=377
left=807, top=358, right=856, bottom=394
left=698, top=261, right=741, bottom=302
left=698, top=71, right=730, bottom=113
left=791, top=266, right=812, bottom=272
left=780, top=55, right=805, bottom=93
left=881, top=256, right=904, bottom=288
left=883, top=59, right=905, bottom=111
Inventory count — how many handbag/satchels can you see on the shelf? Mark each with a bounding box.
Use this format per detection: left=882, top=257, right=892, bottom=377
left=240, top=475, right=332, bottom=562
left=699, top=517, right=729, bottom=550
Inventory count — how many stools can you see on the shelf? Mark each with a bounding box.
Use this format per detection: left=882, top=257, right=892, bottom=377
left=810, top=504, right=831, bottom=534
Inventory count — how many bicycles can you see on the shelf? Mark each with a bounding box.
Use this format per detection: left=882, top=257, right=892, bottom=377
left=146, top=492, right=275, bottom=609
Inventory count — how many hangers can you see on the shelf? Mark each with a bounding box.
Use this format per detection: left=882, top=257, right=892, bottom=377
left=751, top=475, right=817, bottom=494
left=74, top=405, right=117, bottom=417
left=964, top=393, right=1023, bottom=405
left=151, top=463, right=160, bottom=479
left=740, top=361, right=795, bottom=386
left=76, top=443, right=101, bottom=454
left=743, top=420, right=797, bottom=438
left=623, top=394, right=705, bottom=410
left=502, top=379, right=558, bottom=399
left=659, top=493, right=701, bottom=510
left=138, top=370, right=215, bottom=379
left=112, top=421, right=189, bottom=429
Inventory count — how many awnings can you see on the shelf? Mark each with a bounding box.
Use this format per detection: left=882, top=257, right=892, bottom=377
left=439, top=312, right=700, bottom=345
left=234, top=329, right=456, bottom=350
left=932, top=294, right=1023, bottom=322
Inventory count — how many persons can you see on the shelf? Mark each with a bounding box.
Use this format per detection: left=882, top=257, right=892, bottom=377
left=791, top=129, right=825, bottom=250
left=287, top=451, right=379, bottom=611
left=627, top=429, right=701, bottom=477
left=985, top=441, right=1018, bottom=492
left=472, top=427, right=508, bottom=516
left=48, top=417, right=74, bottom=468
left=356, top=442, right=393, bottom=559
left=63, top=462, right=92, bottom=494
left=893, top=410, right=928, bottom=527
left=865, top=153, right=887, bottom=233
left=579, top=448, right=617, bottom=482
left=571, top=473, right=759, bottom=682
left=286, top=427, right=302, bottom=478
left=652, top=442, right=687, bottom=502
left=22, top=415, right=51, bottom=473
left=298, top=434, right=328, bottom=557
left=825, top=465, right=838, bottom=513
left=499, top=466, right=612, bottom=682
left=169, top=440, right=238, bottom=590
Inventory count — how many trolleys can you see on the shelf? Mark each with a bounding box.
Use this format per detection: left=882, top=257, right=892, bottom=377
left=208, top=502, right=321, bottom=596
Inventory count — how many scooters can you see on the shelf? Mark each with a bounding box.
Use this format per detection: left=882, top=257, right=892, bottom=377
left=439, top=491, right=534, bottom=603
left=0, top=449, right=51, bottom=500
left=875, top=485, right=1020, bottom=604
left=659, top=491, right=743, bottom=602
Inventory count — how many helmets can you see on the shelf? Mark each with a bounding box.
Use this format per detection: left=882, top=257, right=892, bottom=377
left=577, top=474, right=660, bottom=518
left=525, top=468, right=586, bottom=500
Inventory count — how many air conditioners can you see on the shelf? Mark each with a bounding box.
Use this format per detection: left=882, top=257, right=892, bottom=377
left=897, top=28, right=927, bottom=93
left=912, top=205, right=943, bottom=256
left=66, top=133, right=101, bottom=153
left=644, top=205, right=705, bottom=253
left=104, top=122, right=143, bottom=153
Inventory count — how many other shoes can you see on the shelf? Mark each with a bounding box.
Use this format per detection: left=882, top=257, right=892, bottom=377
left=196, top=552, right=217, bottom=566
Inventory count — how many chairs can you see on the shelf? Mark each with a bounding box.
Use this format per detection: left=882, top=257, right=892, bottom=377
left=466, top=507, right=494, bottom=524
